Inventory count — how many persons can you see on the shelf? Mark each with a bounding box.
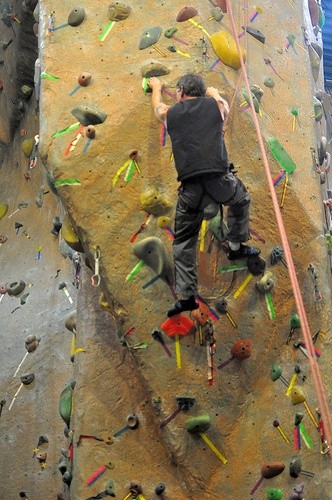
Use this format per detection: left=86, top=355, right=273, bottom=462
left=148, top=72, right=261, bottom=318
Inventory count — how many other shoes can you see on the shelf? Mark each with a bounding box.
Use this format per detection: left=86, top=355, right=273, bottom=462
left=227, top=243, right=260, bottom=261
left=167, top=294, right=200, bottom=318
